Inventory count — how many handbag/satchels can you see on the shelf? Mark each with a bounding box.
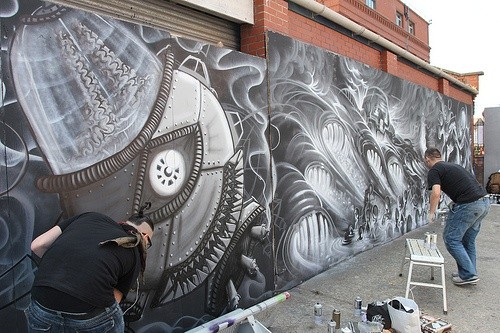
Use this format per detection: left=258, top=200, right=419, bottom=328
left=387, top=288, right=421, bottom=333
left=366, top=300, right=391, bottom=329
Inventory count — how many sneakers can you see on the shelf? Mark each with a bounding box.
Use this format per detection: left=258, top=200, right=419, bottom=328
left=451, top=272, right=480, bottom=285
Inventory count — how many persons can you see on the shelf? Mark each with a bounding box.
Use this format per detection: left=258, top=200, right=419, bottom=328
left=23, top=201, right=154, bottom=333
left=425, top=147, right=490, bottom=286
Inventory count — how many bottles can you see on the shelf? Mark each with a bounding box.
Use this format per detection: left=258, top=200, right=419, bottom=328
left=423, top=232, right=430, bottom=249
left=354, top=296, right=362, bottom=316
left=328, top=319, right=336, bottom=333
left=333, top=308, right=341, bottom=329
left=430, top=232, right=437, bottom=249
left=314, top=302, right=322, bottom=325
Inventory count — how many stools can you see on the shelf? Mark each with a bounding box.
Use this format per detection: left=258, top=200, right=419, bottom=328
left=399, top=239, right=448, bottom=315
